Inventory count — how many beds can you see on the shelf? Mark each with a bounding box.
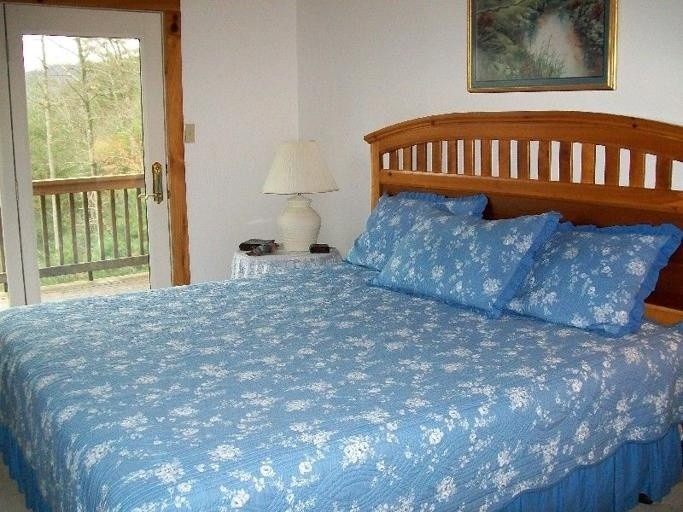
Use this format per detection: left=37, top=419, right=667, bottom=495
left=0, top=110, right=683, bottom=512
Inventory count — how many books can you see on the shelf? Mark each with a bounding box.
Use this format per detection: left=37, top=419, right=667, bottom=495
left=238, top=238, right=275, bottom=251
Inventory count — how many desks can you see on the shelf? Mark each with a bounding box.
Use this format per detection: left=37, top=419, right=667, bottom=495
left=231, top=247, right=338, bottom=281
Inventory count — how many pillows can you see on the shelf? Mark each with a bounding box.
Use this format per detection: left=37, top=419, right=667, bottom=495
left=365, top=211, right=561, bottom=320
left=505, top=221, right=681, bottom=338
left=344, top=190, right=489, bottom=272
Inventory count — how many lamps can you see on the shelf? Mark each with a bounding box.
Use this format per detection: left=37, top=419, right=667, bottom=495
left=259, top=143, right=338, bottom=253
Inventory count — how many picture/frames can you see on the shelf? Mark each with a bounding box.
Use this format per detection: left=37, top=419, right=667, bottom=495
left=466, top=0, right=618, bottom=93
